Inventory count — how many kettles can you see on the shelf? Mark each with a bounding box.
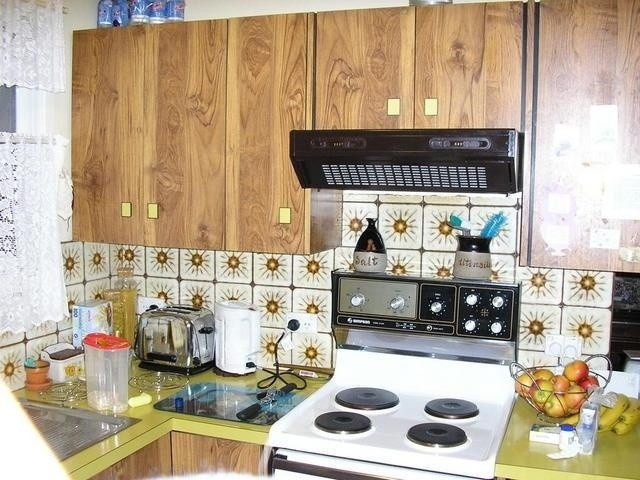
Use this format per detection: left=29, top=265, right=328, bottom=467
left=214, top=302, right=259, bottom=375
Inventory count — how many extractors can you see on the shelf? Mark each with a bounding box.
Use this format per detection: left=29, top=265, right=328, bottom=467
left=287, top=128, right=523, bottom=194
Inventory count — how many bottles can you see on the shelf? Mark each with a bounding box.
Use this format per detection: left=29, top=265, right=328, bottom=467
left=557, top=424, right=576, bottom=450
left=112, top=267, right=137, bottom=349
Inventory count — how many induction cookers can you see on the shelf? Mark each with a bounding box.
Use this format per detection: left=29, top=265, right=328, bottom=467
left=269, top=350, right=518, bottom=479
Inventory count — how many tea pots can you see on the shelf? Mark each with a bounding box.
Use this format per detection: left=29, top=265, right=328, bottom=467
left=86, top=331, right=129, bottom=415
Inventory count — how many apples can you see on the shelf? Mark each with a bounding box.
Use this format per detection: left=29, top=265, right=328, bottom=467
left=515, top=360, right=599, bottom=418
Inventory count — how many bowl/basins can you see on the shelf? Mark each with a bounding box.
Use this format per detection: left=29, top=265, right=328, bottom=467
left=24, top=361, right=51, bottom=384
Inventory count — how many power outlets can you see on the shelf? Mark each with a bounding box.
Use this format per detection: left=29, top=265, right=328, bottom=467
left=286, top=313, right=318, bottom=335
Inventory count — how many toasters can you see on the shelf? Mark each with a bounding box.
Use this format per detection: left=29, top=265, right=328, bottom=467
left=138, top=305, right=213, bottom=377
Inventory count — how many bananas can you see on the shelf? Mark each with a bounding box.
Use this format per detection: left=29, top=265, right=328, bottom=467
left=560, top=391, right=640, bottom=434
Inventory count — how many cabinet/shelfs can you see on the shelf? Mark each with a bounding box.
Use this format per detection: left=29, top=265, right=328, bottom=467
left=88, top=432, right=172, bottom=480
left=315, top=2, right=526, bottom=136
left=171, top=431, right=263, bottom=477
left=226, top=12, right=341, bottom=255
left=521, top=0, right=639, bottom=275
left=73, top=18, right=227, bottom=253
